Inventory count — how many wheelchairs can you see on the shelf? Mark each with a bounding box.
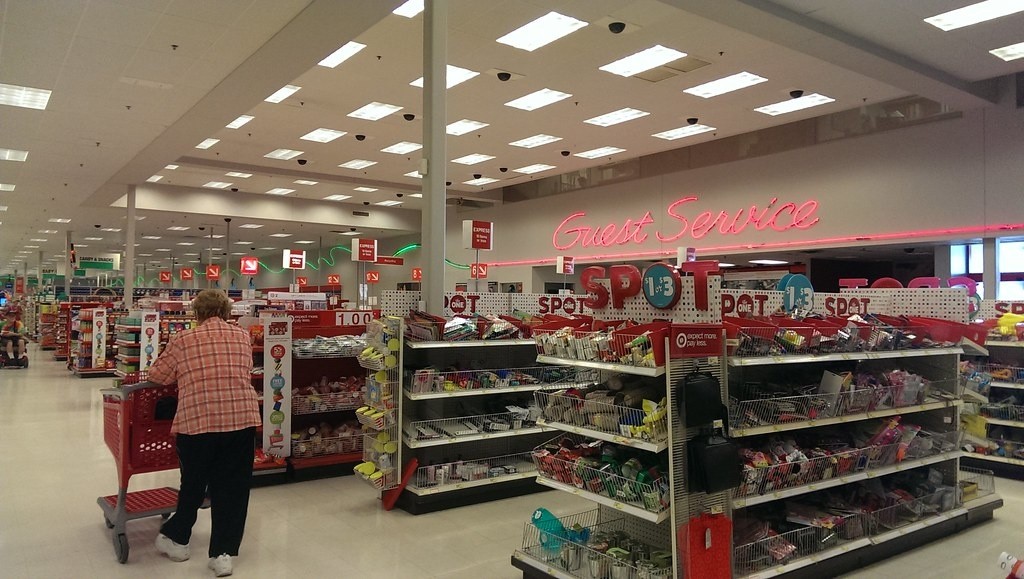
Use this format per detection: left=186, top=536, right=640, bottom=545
left=0, top=337, right=29, bottom=369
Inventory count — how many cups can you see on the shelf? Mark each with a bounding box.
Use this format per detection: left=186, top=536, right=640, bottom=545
left=559, top=530, right=658, bottom=579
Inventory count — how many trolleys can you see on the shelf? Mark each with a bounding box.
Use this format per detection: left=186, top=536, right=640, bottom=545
left=96, top=371, right=210, bottom=564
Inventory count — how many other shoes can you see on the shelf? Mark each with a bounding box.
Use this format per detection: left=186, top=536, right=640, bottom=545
left=11, top=358, right=24, bottom=363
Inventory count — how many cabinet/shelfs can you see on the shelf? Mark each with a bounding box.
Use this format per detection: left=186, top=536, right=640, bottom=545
left=16, top=261, right=1024, bottom=579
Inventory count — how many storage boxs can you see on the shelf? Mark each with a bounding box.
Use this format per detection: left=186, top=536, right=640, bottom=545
left=572, top=320, right=639, bottom=360
left=776, top=314, right=842, bottom=339
left=820, top=318, right=873, bottom=343
left=614, top=322, right=673, bottom=367
left=751, top=317, right=815, bottom=353
left=532, top=318, right=591, bottom=356
left=967, top=322, right=989, bottom=346
left=722, top=316, right=778, bottom=356
left=908, top=315, right=967, bottom=346
left=875, top=315, right=928, bottom=347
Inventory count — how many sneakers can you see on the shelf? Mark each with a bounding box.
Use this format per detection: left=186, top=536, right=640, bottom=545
left=207, top=552, right=232, bottom=577
left=155, top=533, right=190, bottom=561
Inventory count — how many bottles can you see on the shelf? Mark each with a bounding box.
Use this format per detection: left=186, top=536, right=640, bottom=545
left=106, top=306, right=129, bottom=359
left=291, top=375, right=367, bottom=458
left=117, top=289, right=174, bottom=301
left=78, top=309, right=92, bottom=330
left=75, top=332, right=91, bottom=368
left=433, top=373, right=444, bottom=390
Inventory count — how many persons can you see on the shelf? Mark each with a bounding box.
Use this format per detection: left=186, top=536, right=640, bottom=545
left=145, top=290, right=262, bottom=577
left=8, top=300, right=23, bottom=321
left=0, top=310, right=26, bottom=359
